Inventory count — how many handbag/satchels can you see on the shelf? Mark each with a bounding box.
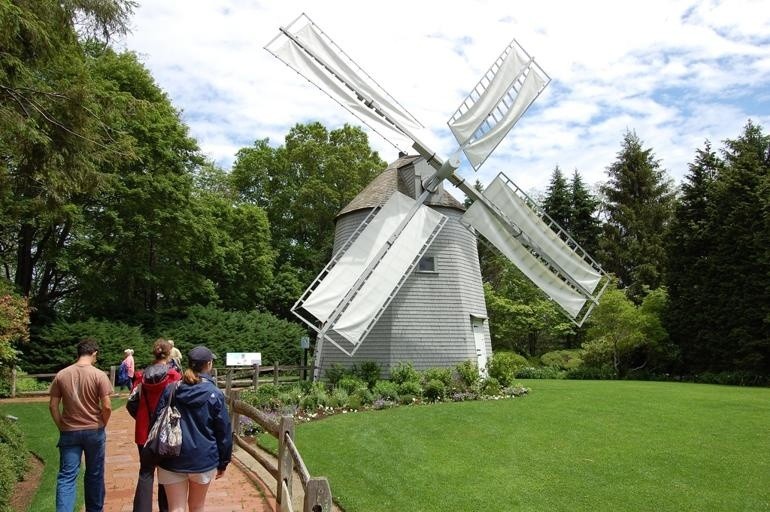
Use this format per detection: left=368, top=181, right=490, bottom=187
left=126, top=383, right=142, bottom=417
left=141, top=406, right=182, bottom=455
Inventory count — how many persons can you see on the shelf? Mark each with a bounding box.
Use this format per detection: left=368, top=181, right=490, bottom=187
left=118, top=337, right=233, bottom=512
left=49, top=340, right=114, bottom=512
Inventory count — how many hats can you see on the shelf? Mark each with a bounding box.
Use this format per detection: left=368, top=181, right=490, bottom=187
left=188, top=346, right=217, bottom=361
